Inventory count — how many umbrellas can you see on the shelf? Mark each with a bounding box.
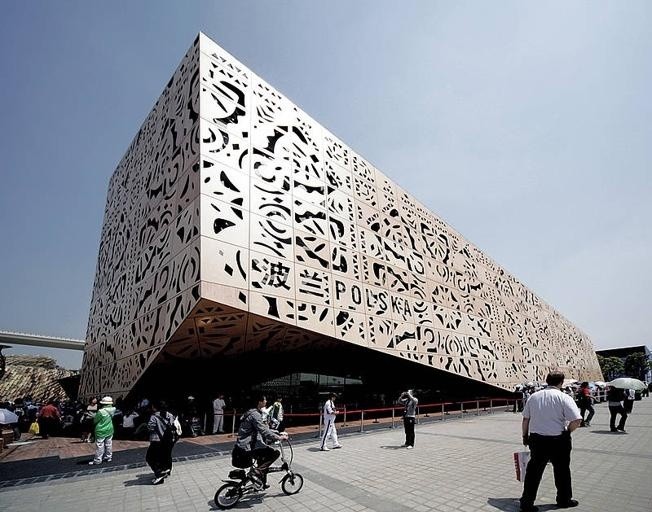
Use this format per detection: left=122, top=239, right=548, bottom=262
left=607, top=378, right=647, bottom=391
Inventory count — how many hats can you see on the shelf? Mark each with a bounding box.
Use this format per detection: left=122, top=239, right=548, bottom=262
left=99, top=397, right=113, bottom=404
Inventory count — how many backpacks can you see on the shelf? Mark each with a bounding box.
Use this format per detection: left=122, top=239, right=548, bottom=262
left=162, top=415, right=183, bottom=449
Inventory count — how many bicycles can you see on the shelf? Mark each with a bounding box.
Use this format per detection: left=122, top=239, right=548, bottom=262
left=210, top=431, right=304, bottom=510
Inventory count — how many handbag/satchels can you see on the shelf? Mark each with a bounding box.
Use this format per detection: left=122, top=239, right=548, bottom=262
left=514, top=445, right=531, bottom=482
left=232, top=444, right=252, bottom=468
left=28, top=422, right=39, bottom=434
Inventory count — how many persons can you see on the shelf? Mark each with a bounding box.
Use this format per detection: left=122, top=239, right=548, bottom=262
left=514, top=384, right=649, bottom=432
left=1, top=392, right=289, bottom=492
left=397, top=388, right=418, bottom=450
left=319, top=392, right=342, bottom=451
left=519, top=371, right=583, bottom=512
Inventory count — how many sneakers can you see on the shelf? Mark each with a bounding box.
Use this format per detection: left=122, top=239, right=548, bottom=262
left=151, top=469, right=170, bottom=484
